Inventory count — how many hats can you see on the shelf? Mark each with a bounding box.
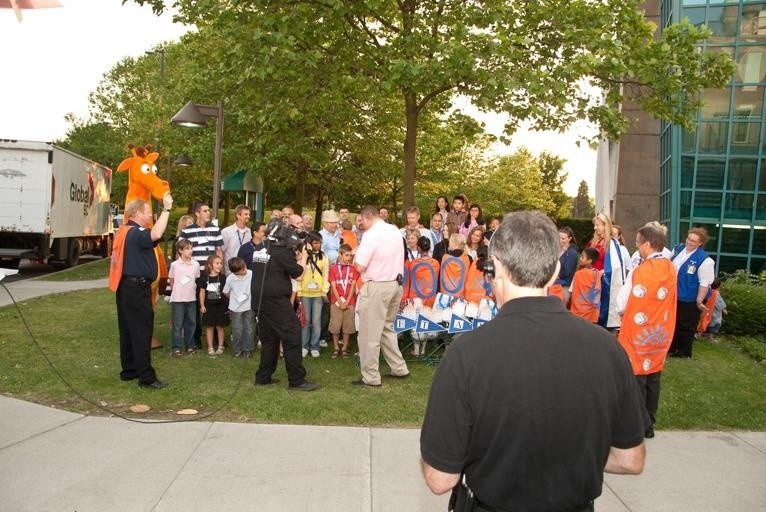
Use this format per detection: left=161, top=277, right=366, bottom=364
left=321, top=209, right=338, bottom=222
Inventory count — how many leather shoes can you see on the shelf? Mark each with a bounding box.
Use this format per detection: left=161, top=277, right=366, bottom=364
left=138, top=378, right=169, bottom=389
left=384, top=372, right=410, bottom=379
left=255, top=378, right=281, bottom=386
left=352, top=379, right=382, bottom=388
left=289, top=382, right=319, bottom=391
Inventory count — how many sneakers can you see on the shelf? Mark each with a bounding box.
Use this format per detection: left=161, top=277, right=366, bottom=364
left=302, top=348, right=308, bottom=358
left=208, top=348, right=216, bottom=356
left=311, top=350, right=320, bottom=358
left=244, top=351, right=253, bottom=360
left=232, top=351, right=242, bottom=360
left=319, top=340, right=328, bottom=347
left=420, top=352, right=425, bottom=358
left=331, top=348, right=340, bottom=358
left=669, top=353, right=692, bottom=358
left=185, top=348, right=197, bottom=354
left=171, top=350, right=182, bottom=358
left=410, top=351, right=420, bottom=358
left=340, top=347, right=350, bottom=359
left=216, top=347, right=225, bottom=355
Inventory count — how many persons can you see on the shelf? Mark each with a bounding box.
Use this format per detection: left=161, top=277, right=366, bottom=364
left=430, top=213, right=444, bottom=246
left=440, top=234, right=473, bottom=347
left=223, top=258, right=253, bottom=359
left=409, top=237, right=439, bottom=358
left=338, top=207, right=356, bottom=232
left=586, top=212, right=626, bottom=331
left=170, top=239, right=201, bottom=356
left=483, top=231, right=493, bottom=246
left=465, top=245, right=494, bottom=304
left=221, top=205, right=253, bottom=277
left=406, top=228, right=422, bottom=262
left=341, top=220, right=358, bottom=249
left=432, top=222, right=459, bottom=264
left=200, top=254, right=227, bottom=356
left=282, top=216, right=290, bottom=225
left=328, top=244, right=360, bottom=360
left=352, top=212, right=364, bottom=243
left=351, top=207, right=410, bottom=387
left=459, top=203, right=486, bottom=236
left=399, top=205, right=434, bottom=258
left=318, top=209, right=344, bottom=348
left=446, top=195, right=468, bottom=231
left=289, top=214, right=304, bottom=230
left=549, top=226, right=578, bottom=305
left=706, top=278, right=728, bottom=334
left=627, top=222, right=673, bottom=274
left=466, top=227, right=485, bottom=251
left=436, top=196, right=450, bottom=225
left=302, top=215, right=313, bottom=233
left=172, top=215, right=195, bottom=261
left=420, top=210, right=653, bottom=511
left=238, top=222, right=266, bottom=270
left=379, top=208, right=393, bottom=225
left=282, top=206, right=294, bottom=216
left=297, top=231, right=329, bottom=358
left=403, top=237, right=409, bottom=261
left=611, top=225, right=631, bottom=274
left=116, top=192, right=173, bottom=390
left=568, top=248, right=601, bottom=322
left=489, top=218, right=500, bottom=232
left=271, top=209, right=282, bottom=218
left=178, top=203, right=229, bottom=350
left=251, top=218, right=320, bottom=392
left=616, top=226, right=676, bottom=438
left=669, top=228, right=714, bottom=358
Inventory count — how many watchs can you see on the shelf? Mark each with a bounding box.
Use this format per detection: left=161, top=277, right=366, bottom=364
left=162, top=208, right=171, bottom=213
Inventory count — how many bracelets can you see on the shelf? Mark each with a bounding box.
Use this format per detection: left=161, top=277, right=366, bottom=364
left=697, top=303, right=702, bottom=305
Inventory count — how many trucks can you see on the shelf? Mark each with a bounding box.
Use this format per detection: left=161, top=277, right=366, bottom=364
left=0, top=139, right=119, bottom=271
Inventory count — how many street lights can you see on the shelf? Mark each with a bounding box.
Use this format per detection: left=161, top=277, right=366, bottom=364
left=143, top=48, right=166, bottom=216
left=171, top=100, right=224, bottom=222
left=174, top=154, right=193, bottom=166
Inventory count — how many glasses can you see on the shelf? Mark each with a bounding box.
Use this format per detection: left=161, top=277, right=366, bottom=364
left=200, top=209, right=210, bottom=212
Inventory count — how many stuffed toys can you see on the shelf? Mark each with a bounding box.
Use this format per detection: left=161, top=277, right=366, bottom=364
left=118, top=144, right=170, bottom=349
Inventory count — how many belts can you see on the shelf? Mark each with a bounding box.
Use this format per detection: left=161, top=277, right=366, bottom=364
left=121, top=275, right=151, bottom=287
